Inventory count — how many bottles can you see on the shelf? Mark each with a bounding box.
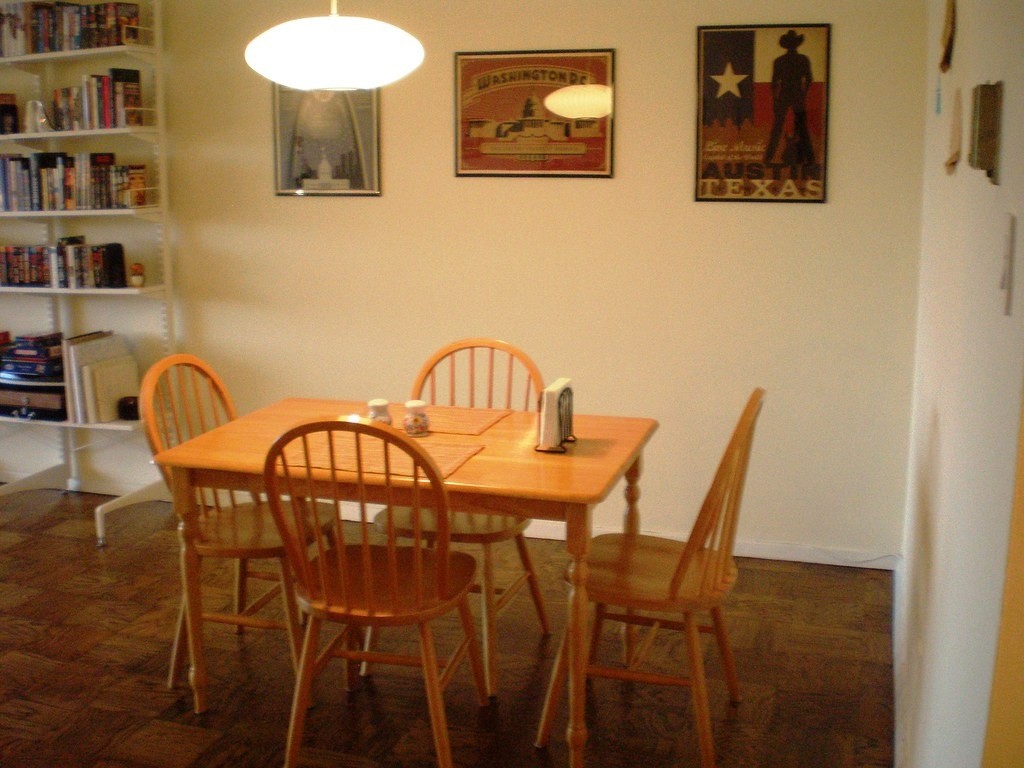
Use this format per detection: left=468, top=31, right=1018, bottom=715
left=404, top=399, right=429, bottom=437
left=366, top=398, right=392, bottom=427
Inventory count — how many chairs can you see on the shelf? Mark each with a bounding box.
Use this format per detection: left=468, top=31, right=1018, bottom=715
left=138, top=352, right=366, bottom=715
left=264, top=416, right=489, bottom=768
left=533, top=387, right=766, bottom=768
left=358, top=337, right=550, bottom=698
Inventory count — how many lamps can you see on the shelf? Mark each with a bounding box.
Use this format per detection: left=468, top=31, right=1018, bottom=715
left=543, top=61, right=612, bottom=117
left=244, top=0, right=425, bottom=92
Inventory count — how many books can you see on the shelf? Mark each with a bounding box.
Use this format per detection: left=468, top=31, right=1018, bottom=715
left=0, top=0, right=140, bottom=57
left=1, top=325, right=143, bottom=425
left=0, top=149, right=147, bottom=212
left=0, top=236, right=127, bottom=290
left=0, top=68, right=142, bottom=134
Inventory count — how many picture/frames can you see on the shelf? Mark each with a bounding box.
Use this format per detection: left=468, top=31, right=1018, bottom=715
left=272, top=82, right=381, bottom=197
left=694, top=23, right=830, bottom=204
left=454, top=49, right=616, bottom=178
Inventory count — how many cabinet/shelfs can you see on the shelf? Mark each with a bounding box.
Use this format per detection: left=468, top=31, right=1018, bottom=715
left=0, top=0, right=180, bottom=547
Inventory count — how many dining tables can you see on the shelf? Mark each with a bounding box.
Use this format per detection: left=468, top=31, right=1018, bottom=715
left=151, top=398, right=658, bottom=768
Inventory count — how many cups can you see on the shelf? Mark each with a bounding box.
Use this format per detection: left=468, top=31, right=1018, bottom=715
left=130, top=269, right=145, bottom=288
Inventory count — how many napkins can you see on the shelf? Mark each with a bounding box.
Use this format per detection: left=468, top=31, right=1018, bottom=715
left=538, top=376, right=570, bottom=448
